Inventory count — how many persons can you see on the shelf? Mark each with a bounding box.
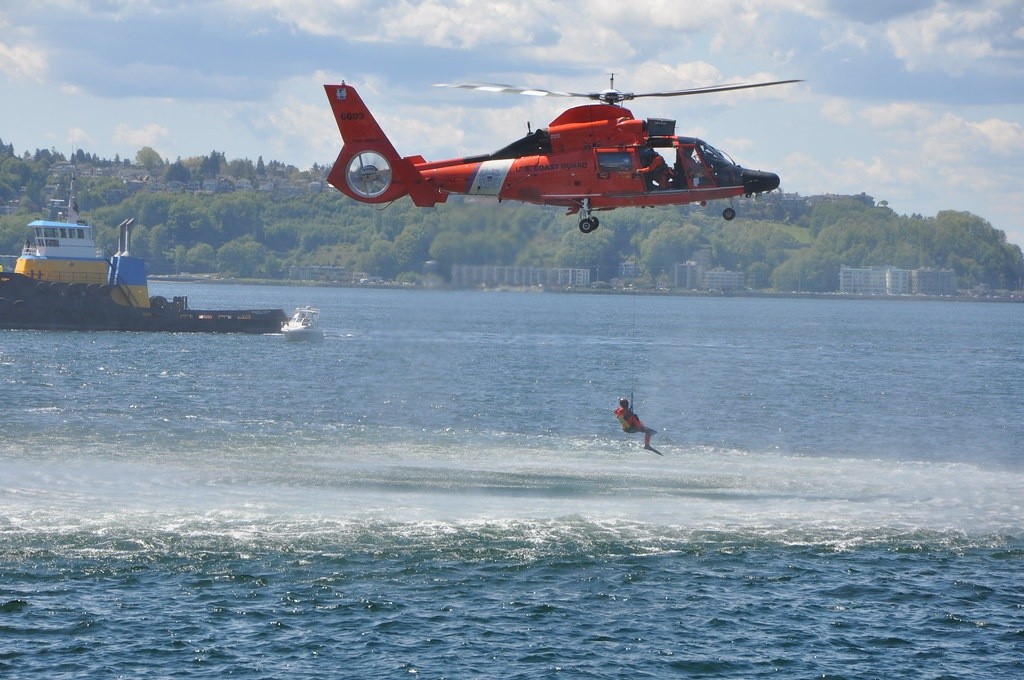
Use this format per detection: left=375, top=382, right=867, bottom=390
left=616, top=398, right=664, bottom=456
left=682, top=147, right=704, bottom=176
left=637, top=147, right=674, bottom=188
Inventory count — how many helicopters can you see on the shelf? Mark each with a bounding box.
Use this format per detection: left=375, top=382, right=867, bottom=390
left=321, top=74, right=806, bottom=233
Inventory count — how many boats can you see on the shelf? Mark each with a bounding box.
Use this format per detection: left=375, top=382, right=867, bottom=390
left=0, top=169, right=288, bottom=335
left=280, top=306, right=325, bottom=340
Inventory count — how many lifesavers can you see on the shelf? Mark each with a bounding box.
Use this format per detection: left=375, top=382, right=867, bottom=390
left=86, top=283, right=99, bottom=296
left=99, top=284, right=110, bottom=296
left=32, top=281, right=82, bottom=297
left=9, top=299, right=139, bottom=326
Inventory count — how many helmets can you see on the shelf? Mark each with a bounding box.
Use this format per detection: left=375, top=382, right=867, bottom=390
left=620, top=399, right=628, bottom=406
